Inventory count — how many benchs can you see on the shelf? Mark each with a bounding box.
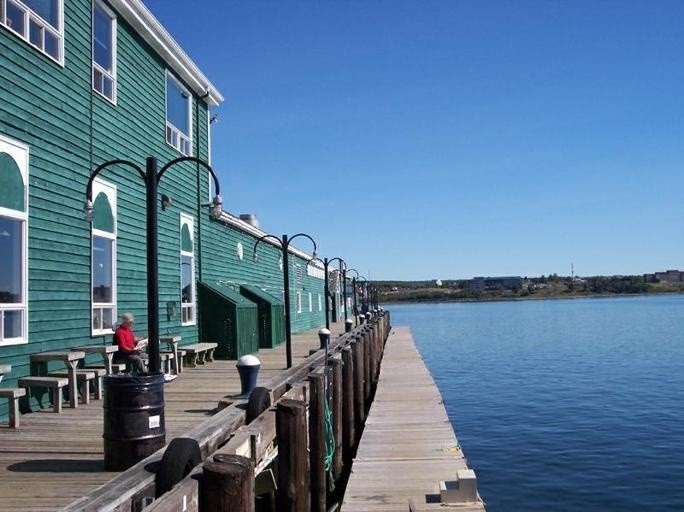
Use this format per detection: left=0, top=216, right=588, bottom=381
left=178, top=342, right=218, bottom=367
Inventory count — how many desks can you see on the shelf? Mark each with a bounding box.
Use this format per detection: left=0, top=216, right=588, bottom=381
left=0, top=335, right=186, bottom=428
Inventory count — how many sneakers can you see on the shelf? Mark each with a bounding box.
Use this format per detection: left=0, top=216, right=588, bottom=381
left=163, top=374, right=178, bottom=382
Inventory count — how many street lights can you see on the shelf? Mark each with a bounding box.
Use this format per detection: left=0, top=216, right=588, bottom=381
left=305, top=256, right=380, bottom=330
left=84, top=156, right=223, bottom=373
left=253, top=232, right=318, bottom=368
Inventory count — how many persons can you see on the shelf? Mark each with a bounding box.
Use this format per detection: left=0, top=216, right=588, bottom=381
left=113, top=313, right=149, bottom=375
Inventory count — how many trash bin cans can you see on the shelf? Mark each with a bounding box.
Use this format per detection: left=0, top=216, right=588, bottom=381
left=102, top=371, right=166, bottom=472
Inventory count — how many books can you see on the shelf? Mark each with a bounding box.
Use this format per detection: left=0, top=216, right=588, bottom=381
left=134, top=336, right=161, bottom=349
